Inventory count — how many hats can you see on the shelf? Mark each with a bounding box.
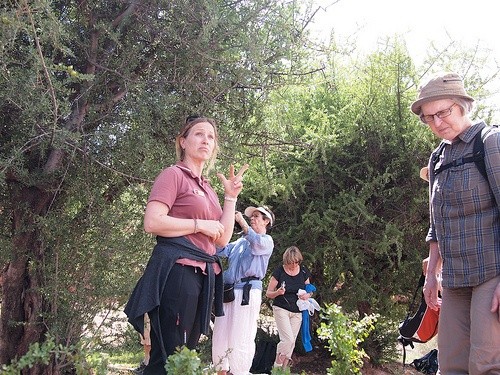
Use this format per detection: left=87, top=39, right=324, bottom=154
left=244, top=206, right=275, bottom=226
left=420, top=166, right=429, bottom=183
left=410, top=73, right=475, bottom=115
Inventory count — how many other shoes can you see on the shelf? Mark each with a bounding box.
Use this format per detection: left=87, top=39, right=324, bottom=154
left=133, top=362, right=147, bottom=375
left=274, top=361, right=283, bottom=369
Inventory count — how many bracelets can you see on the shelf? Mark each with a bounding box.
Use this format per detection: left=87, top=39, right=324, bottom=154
left=224, top=196, right=237, bottom=202
left=193, top=217, right=197, bottom=234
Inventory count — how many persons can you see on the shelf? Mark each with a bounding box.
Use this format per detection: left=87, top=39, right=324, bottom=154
left=212, top=205, right=275, bottom=375
left=411, top=74, right=500, bottom=375
left=266, top=246, right=312, bottom=368
left=125, top=117, right=249, bottom=375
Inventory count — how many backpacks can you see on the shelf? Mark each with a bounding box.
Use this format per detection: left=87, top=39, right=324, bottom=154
left=397, top=271, right=443, bottom=366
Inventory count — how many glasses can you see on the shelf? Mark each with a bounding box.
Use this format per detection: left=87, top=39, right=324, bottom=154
left=419, top=103, right=456, bottom=122
